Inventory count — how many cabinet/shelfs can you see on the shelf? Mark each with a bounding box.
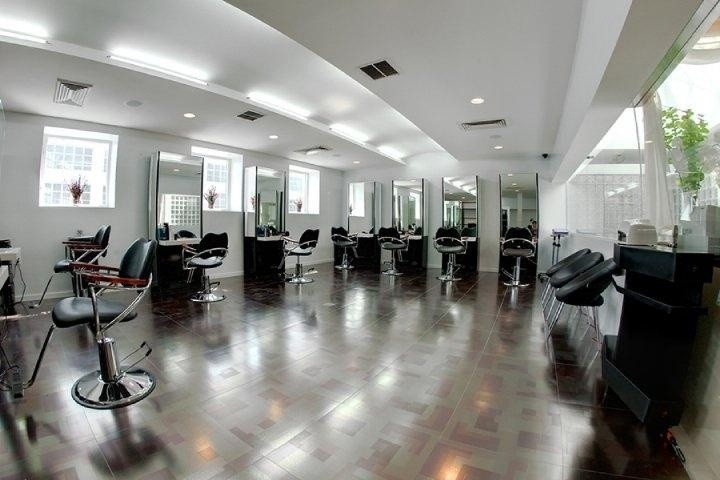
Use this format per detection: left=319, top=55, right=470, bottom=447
left=602, top=238, right=719, bottom=432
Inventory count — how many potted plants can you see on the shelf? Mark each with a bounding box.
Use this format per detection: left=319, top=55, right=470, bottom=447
left=657, top=105, right=710, bottom=220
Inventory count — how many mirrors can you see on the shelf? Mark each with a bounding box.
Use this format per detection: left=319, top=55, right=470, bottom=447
left=256, top=166, right=286, bottom=234
left=155, top=150, right=205, bottom=239
left=347, top=176, right=540, bottom=240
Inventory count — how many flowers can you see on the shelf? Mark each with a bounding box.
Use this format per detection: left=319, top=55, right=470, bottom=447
left=250, top=195, right=255, bottom=209
left=66, top=175, right=89, bottom=197
left=202, top=185, right=219, bottom=204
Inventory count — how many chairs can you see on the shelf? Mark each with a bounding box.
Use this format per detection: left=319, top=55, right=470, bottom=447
left=257, top=225, right=278, bottom=234
left=11, top=235, right=160, bottom=417
left=538, top=247, right=621, bottom=345
left=173, top=229, right=197, bottom=239
left=327, top=225, right=537, bottom=288
left=278, top=228, right=322, bottom=286
left=28, top=223, right=113, bottom=316
left=179, top=231, right=230, bottom=305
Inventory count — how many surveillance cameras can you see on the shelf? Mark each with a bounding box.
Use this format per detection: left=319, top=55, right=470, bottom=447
left=542, top=152, right=549, bottom=159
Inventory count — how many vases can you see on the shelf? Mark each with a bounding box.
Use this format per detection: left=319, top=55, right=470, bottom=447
left=73, top=196, right=83, bottom=205
left=208, top=203, right=213, bottom=208
